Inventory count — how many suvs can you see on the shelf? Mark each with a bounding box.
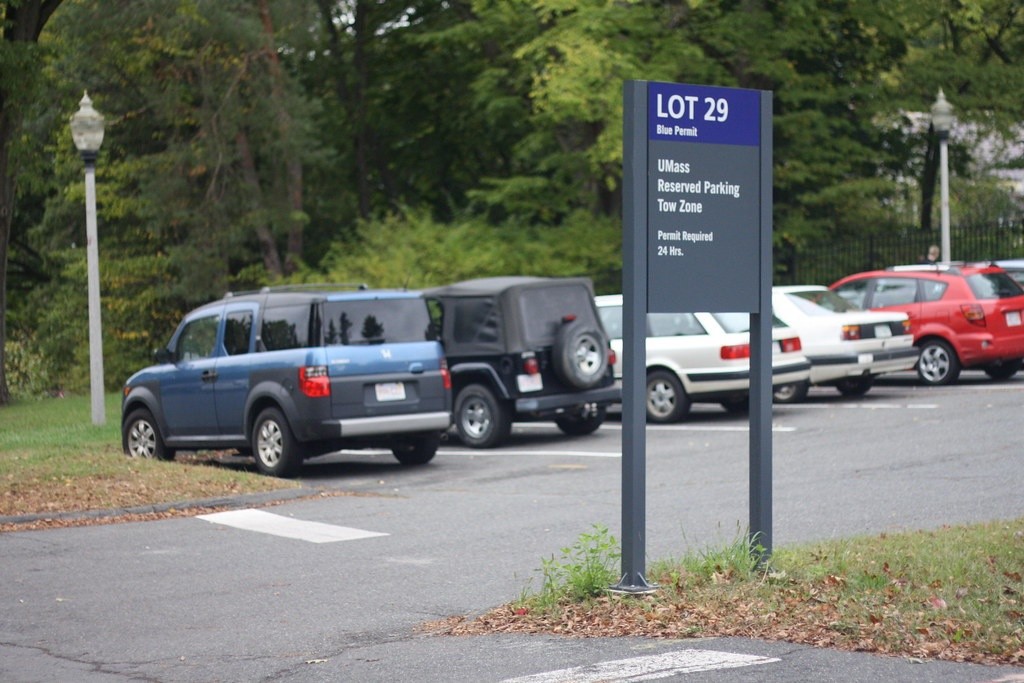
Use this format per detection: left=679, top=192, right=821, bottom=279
left=424, top=273, right=625, bottom=448
left=119, top=285, right=455, bottom=479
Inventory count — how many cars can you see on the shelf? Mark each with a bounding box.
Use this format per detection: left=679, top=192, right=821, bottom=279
left=586, top=284, right=815, bottom=424
left=776, top=281, right=922, bottom=406
left=823, top=261, right=1024, bottom=386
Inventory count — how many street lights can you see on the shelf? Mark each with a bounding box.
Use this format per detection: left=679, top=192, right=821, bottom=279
left=68, top=88, right=106, bottom=427
left=929, top=88, right=956, bottom=263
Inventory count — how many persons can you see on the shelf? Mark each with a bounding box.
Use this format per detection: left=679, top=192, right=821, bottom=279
left=920, top=245, right=939, bottom=264
